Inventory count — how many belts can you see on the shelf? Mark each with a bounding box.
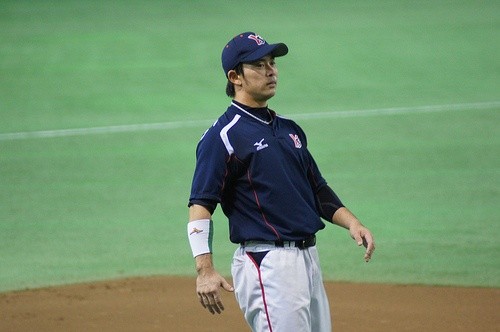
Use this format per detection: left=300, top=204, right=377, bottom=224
left=275, top=234, right=316, bottom=250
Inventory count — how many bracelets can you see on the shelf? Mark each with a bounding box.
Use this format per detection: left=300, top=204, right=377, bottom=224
left=187, top=219, right=214, bottom=258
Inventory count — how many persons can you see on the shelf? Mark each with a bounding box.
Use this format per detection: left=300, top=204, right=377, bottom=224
left=186, top=32, right=376, bottom=332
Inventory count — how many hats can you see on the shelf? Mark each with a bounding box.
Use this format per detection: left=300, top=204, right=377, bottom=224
left=221, top=31, right=288, bottom=78
